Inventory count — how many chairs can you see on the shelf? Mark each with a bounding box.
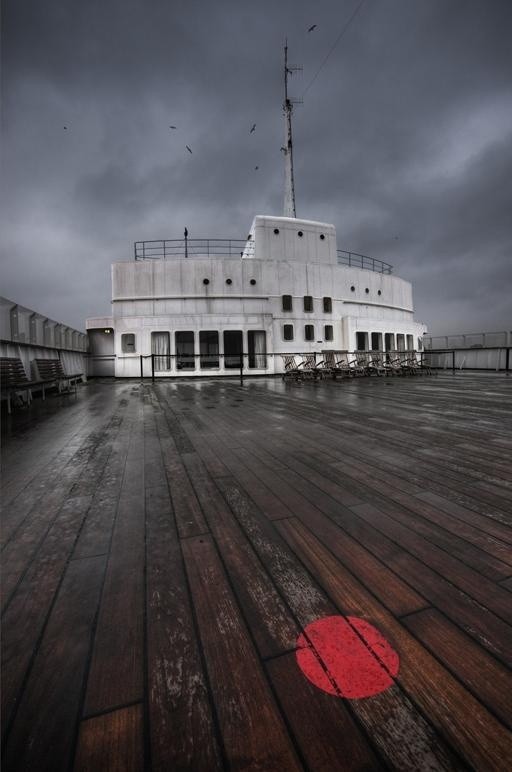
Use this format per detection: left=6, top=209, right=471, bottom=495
left=280, top=350, right=436, bottom=384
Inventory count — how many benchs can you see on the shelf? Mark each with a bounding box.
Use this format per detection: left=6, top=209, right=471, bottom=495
left=35, top=358, right=84, bottom=400
left=1, top=355, right=56, bottom=414
